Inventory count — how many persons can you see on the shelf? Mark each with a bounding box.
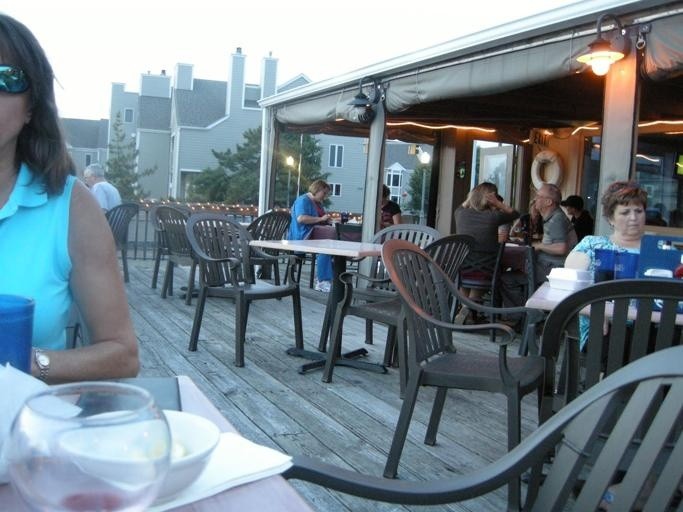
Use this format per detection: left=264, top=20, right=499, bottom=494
left=0, top=14, right=140, bottom=384
left=509, top=198, right=542, bottom=242
left=564, top=182, right=682, bottom=375
left=499, top=184, right=579, bottom=330
left=379, top=184, right=402, bottom=227
left=454, top=182, right=519, bottom=328
left=557, top=193, right=593, bottom=243
left=285, top=180, right=333, bottom=294
left=83, top=163, right=123, bottom=216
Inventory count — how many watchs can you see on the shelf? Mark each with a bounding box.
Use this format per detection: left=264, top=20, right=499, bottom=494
left=34, top=346, right=50, bottom=381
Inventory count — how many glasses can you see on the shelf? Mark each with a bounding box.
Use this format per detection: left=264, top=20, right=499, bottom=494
left=0, top=64, right=31, bottom=95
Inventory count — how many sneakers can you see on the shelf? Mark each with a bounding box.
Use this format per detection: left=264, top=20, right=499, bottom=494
left=454, top=307, right=476, bottom=324
left=314, top=280, right=331, bottom=293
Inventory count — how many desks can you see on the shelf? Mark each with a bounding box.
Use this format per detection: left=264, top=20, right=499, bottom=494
left=524, top=269, right=682, bottom=446
left=1, top=375, right=315, bottom=512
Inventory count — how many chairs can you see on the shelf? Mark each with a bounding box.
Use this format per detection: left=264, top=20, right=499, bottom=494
left=102, top=204, right=593, bottom=383
left=380, top=238, right=551, bottom=512
left=274, top=343, right=683, bottom=512
left=520, top=276, right=682, bottom=510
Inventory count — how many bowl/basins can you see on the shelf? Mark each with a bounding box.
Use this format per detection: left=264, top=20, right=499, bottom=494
left=64, top=410, right=220, bottom=505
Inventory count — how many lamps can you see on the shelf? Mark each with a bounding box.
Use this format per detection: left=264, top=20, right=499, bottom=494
left=347, top=75, right=380, bottom=106
left=575, top=9, right=628, bottom=76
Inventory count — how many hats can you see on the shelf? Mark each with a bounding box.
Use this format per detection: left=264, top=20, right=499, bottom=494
left=560, top=195, right=584, bottom=210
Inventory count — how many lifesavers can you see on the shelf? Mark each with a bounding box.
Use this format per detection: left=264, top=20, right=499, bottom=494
left=531, top=151, right=563, bottom=190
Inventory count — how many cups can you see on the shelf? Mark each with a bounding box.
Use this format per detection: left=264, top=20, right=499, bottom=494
left=5, top=380, right=171, bottom=512
left=595, top=248, right=638, bottom=281
left=0, top=295, right=36, bottom=373
